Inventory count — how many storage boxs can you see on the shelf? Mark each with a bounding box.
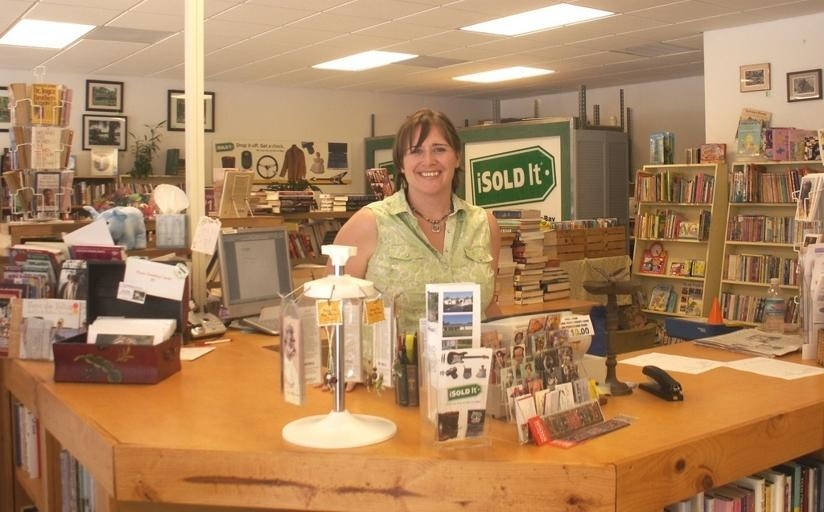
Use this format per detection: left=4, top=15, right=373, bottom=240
left=52, top=258, right=190, bottom=385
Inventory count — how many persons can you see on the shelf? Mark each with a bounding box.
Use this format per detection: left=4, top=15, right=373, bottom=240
left=316, top=107, right=502, bottom=394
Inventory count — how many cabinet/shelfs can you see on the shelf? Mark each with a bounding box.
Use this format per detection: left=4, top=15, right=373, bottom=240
left=0, top=358, right=116, bottom=511
left=278, top=213, right=355, bottom=285
left=632, top=164, right=727, bottom=318
left=792, top=188, right=824, bottom=363
left=8, top=85, right=75, bottom=222
left=718, top=160, right=824, bottom=327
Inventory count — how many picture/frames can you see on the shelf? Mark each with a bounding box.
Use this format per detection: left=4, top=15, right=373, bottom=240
left=82, top=114, right=128, bottom=152
left=740, top=63, right=771, bottom=92
left=167, top=90, right=215, bottom=132
left=0, top=87, right=9, bottom=132
left=86, top=80, right=123, bottom=111
left=787, top=69, right=822, bottom=102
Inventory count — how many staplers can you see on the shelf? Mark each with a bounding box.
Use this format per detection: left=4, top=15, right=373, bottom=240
left=639, top=365, right=683, bottom=401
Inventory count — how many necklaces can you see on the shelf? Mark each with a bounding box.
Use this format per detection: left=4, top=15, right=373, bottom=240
left=409, top=205, right=451, bottom=234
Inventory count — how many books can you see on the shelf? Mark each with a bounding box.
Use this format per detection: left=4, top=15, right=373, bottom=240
left=661, top=458, right=823, bottom=512
left=100, top=174, right=315, bottom=262
left=638, top=208, right=710, bottom=241
left=494, top=210, right=625, bottom=305
left=736, top=110, right=818, bottom=160
left=0, top=74, right=93, bottom=511
left=318, top=159, right=393, bottom=213
left=732, top=164, right=818, bottom=204
left=635, top=170, right=713, bottom=203
left=729, top=253, right=799, bottom=286
left=721, top=293, right=800, bottom=325
left=649, top=285, right=702, bottom=314
left=730, top=215, right=794, bottom=244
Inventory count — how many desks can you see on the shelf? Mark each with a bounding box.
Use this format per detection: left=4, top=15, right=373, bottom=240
left=9, top=220, right=93, bottom=248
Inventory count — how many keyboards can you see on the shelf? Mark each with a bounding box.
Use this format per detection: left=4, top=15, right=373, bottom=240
left=242, top=315, right=282, bottom=335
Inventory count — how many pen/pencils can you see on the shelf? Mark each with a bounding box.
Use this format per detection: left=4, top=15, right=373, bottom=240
left=198, top=338, right=233, bottom=345
left=396, top=332, right=419, bottom=406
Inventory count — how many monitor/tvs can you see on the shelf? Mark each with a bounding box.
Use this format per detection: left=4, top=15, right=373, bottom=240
left=217, top=225, right=292, bottom=319
